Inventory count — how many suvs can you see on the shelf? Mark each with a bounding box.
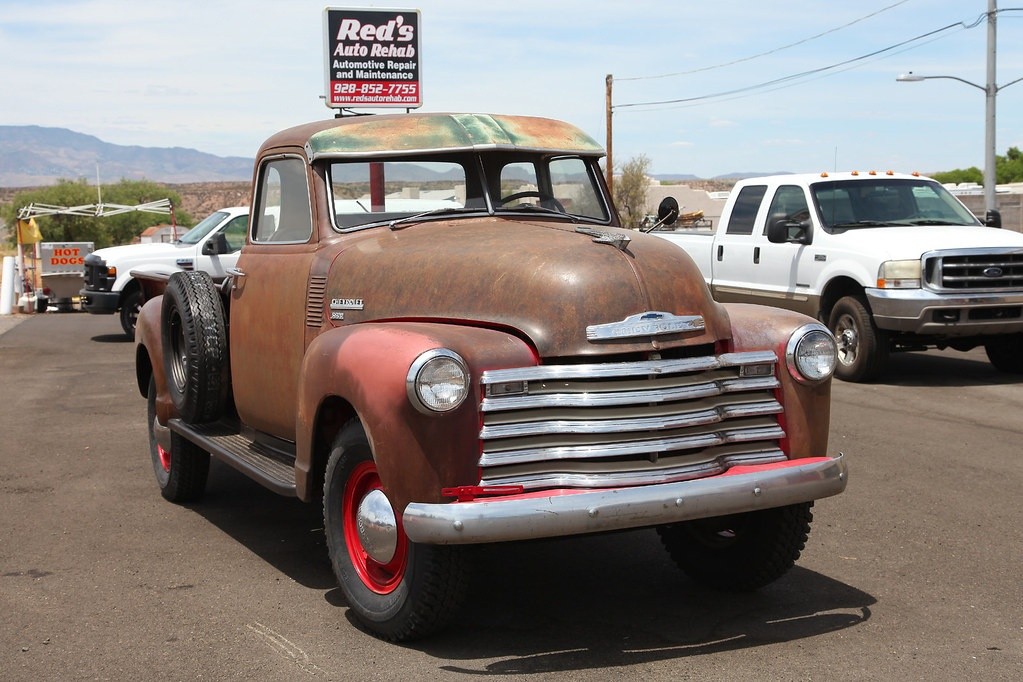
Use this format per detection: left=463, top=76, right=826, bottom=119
left=78, top=198, right=463, bottom=343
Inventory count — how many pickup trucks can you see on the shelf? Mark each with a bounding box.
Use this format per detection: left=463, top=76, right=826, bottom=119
left=630, top=169, right=1023, bottom=383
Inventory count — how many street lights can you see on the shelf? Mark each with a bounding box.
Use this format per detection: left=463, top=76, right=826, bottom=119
left=896, top=71, right=1023, bottom=226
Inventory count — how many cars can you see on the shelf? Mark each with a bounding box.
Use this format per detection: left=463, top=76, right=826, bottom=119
left=133, top=113, right=849, bottom=648
